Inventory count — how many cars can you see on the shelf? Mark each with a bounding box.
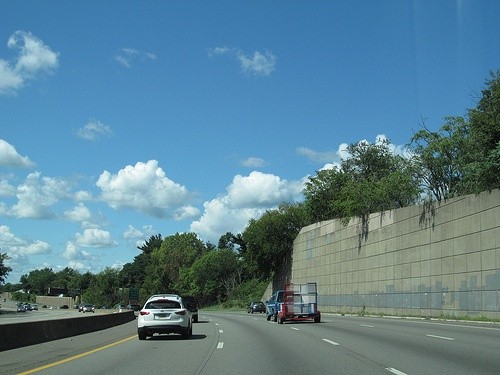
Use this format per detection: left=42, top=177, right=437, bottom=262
left=247, top=301, right=266, bottom=314
left=93, top=304, right=141, bottom=311
left=82, top=303, right=95, bottom=313
left=41, top=303, right=83, bottom=312
left=15, top=302, right=39, bottom=313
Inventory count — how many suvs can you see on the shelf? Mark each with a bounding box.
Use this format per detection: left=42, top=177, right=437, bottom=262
left=137, top=293, right=193, bottom=340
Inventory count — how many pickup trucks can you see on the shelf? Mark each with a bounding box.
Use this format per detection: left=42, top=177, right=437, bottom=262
left=265, top=289, right=304, bottom=324
left=180, top=295, right=198, bottom=323
